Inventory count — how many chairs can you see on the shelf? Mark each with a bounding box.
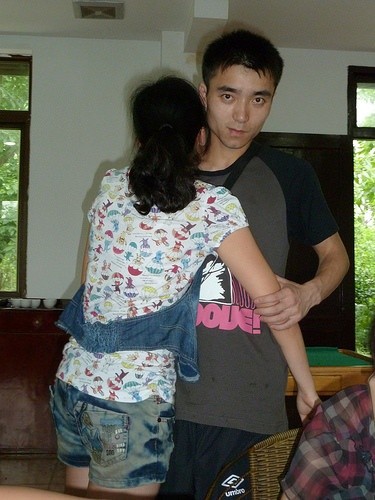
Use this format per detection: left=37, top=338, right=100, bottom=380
left=204, top=428, right=303, bottom=500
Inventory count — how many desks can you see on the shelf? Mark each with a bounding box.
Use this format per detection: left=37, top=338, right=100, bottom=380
left=285, top=347, right=375, bottom=397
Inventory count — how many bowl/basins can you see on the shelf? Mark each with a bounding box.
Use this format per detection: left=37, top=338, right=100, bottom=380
left=21, top=299, right=31, bottom=308
left=11, top=299, right=22, bottom=308
left=43, top=300, right=57, bottom=309
left=31, top=299, right=41, bottom=308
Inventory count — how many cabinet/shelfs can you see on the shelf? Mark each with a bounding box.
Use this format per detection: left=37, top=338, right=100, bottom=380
left=0, top=299, right=79, bottom=461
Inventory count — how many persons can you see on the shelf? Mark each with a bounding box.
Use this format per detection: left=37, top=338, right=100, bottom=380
left=157, top=30, right=351, bottom=497
left=50, top=75, right=323, bottom=500
left=275, top=313, right=375, bottom=500
